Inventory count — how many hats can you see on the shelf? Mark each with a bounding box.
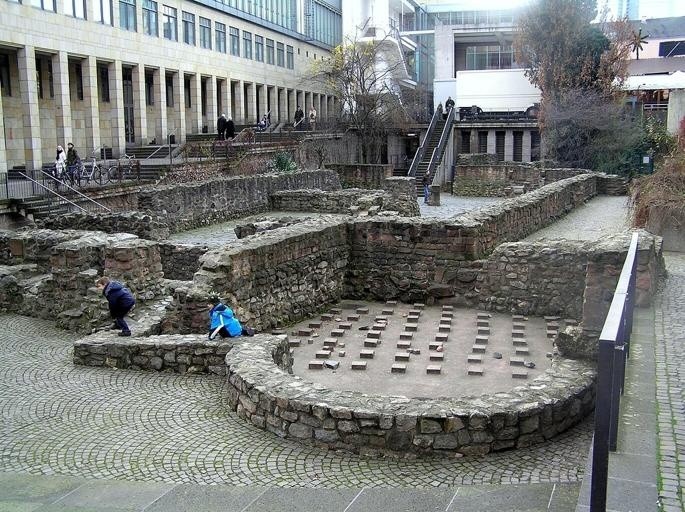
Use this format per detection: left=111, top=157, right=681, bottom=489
left=207, top=295, right=221, bottom=304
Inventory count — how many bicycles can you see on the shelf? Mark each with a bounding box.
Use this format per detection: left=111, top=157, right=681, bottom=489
left=73, top=155, right=111, bottom=188
left=108, top=152, right=140, bottom=183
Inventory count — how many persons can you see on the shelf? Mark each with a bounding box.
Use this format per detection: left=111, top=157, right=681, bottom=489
left=54, top=145, right=67, bottom=179
left=207, top=295, right=257, bottom=341
left=67, top=143, right=80, bottom=184
left=294, top=105, right=304, bottom=126
left=97, top=275, right=134, bottom=336
left=217, top=113, right=226, bottom=141
left=308, top=106, right=317, bottom=131
left=445, top=96, right=455, bottom=114
left=225, top=117, right=235, bottom=139
left=421, top=170, right=432, bottom=202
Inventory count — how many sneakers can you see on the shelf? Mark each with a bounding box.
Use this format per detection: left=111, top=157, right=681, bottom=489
left=118, top=331, right=131, bottom=337
left=111, top=324, right=122, bottom=330
